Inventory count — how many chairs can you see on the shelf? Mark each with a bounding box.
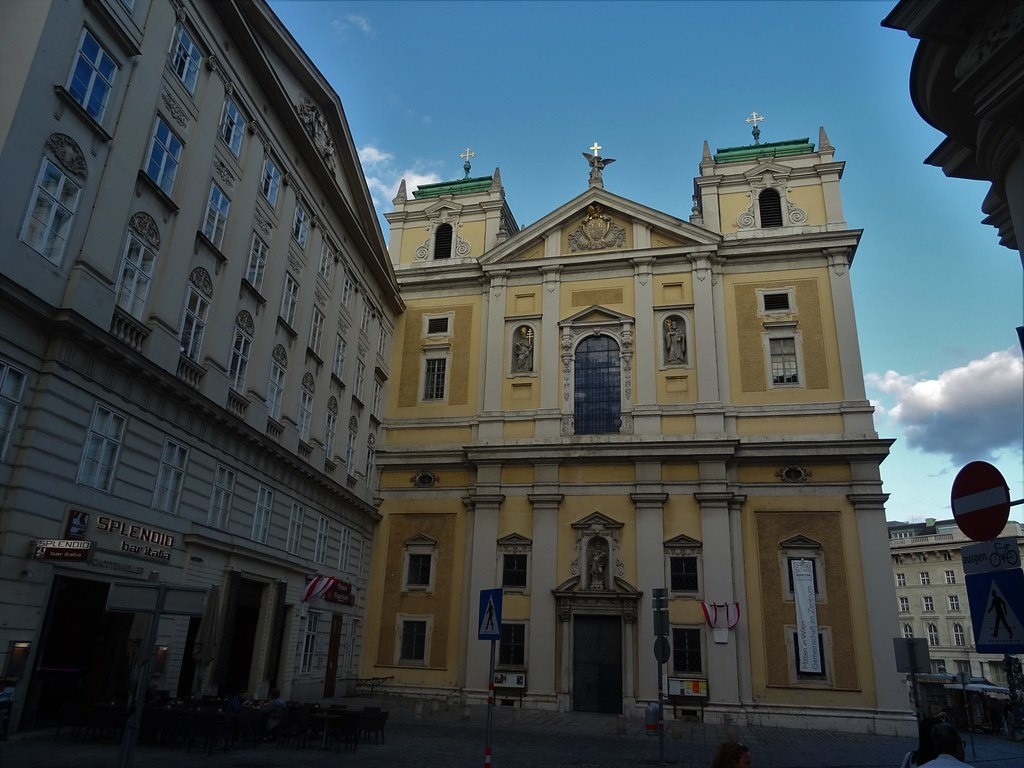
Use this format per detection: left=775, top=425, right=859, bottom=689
left=54, top=689, right=388, bottom=757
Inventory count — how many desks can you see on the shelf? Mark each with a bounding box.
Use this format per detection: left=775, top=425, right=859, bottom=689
left=305, top=713, right=345, bottom=752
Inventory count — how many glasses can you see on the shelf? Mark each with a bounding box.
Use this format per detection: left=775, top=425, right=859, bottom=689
left=960, top=740, right=966, bottom=749
left=735, top=741, right=743, bottom=754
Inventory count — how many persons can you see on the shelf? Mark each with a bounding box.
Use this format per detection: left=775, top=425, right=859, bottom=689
left=918, top=723, right=975, bottom=768
left=713, top=741, right=751, bottom=768
left=516, top=333, right=532, bottom=372
left=225, top=689, right=286, bottom=743
left=991, top=698, right=1009, bottom=736
left=899, top=717, right=945, bottom=768
left=665, top=322, right=685, bottom=363
left=589, top=156, right=604, bottom=178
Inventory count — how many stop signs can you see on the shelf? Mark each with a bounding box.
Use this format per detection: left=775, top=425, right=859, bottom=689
left=950, top=459, right=1011, bottom=541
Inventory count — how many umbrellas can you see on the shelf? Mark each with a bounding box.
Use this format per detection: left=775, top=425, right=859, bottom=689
left=128, top=571, right=159, bottom=665
left=193, top=584, right=219, bottom=696
